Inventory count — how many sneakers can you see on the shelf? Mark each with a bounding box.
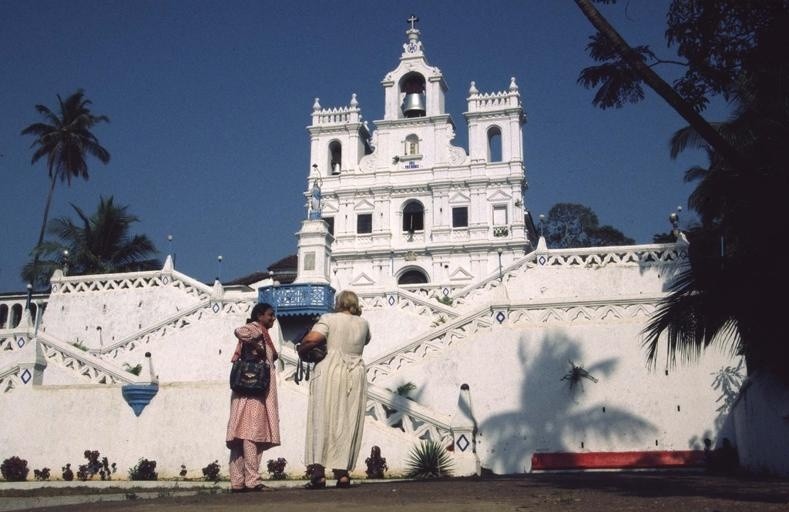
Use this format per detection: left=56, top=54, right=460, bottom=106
left=232, top=483, right=274, bottom=492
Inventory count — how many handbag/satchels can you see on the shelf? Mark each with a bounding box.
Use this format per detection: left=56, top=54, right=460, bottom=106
left=296, top=326, right=327, bottom=362
left=229, top=333, right=270, bottom=395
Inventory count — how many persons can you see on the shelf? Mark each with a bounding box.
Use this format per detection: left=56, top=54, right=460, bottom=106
left=295, top=289, right=374, bottom=491
left=212, top=277, right=223, bottom=287
left=223, top=300, right=283, bottom=493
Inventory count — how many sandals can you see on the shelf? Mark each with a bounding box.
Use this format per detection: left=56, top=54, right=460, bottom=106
left=304, top=474, right=351, bottom=489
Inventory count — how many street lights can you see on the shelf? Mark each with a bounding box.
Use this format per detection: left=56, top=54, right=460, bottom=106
left=168, top=235, right=173, bottom=256
left=333, top=267, right=337, bottom=275
left=677, top=206, right=682, bottom=233
left=63, top=250, right=70, bottom=274
left=497, top=247, right=503, bottom=284
left=216, top=255, right=223, bottom=280
left=539, top=214, right=545, bottom=236
left=25, top=283, right=34, bottom=310
left=269, top=270, right=274, bottom=280
